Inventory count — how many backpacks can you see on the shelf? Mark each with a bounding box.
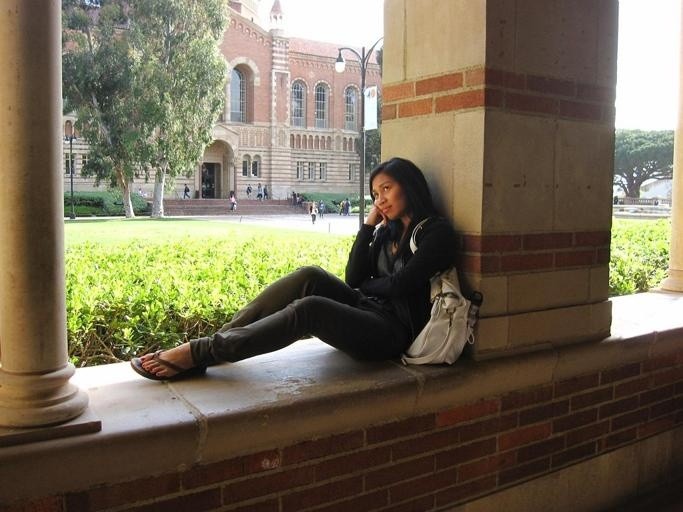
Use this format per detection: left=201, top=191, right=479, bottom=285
left=399, top=213, right=483, bottom=367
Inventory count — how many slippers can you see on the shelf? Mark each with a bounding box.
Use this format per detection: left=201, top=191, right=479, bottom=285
left=129, top=348, right=208, bottom=382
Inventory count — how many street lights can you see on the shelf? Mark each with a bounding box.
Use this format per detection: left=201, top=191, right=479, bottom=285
left=335, top=36, right=382, bottom=229
left=64, top=134, right=77, bottom=220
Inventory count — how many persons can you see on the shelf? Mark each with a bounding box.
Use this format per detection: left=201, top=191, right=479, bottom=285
left=613, top=195, right=619, bottom=205
left=342, top=198, right=351, bottom=217
left=228, top=192, right=237, bottom=213
left=291, top=191, right=297, bottom=205
left=296, top=192, right=301, bottom=204
left=339, top=199, right=345, bottom=215
left=138, top=188, right=146, bottom=199
left=257, top=183, right=263, bottom=203
left=129, top=155, right=458, bottom=381
left=654, top=198, right=658, bottom=206
left=319, top=199, right=325, bottom=218
left=183, top=184, right=191, bottom=200
left=263, top=184, right=269, bottom=200
left=246, top=184, right=253, bottom=199
left=311, top=202, right=319, bottom=225
